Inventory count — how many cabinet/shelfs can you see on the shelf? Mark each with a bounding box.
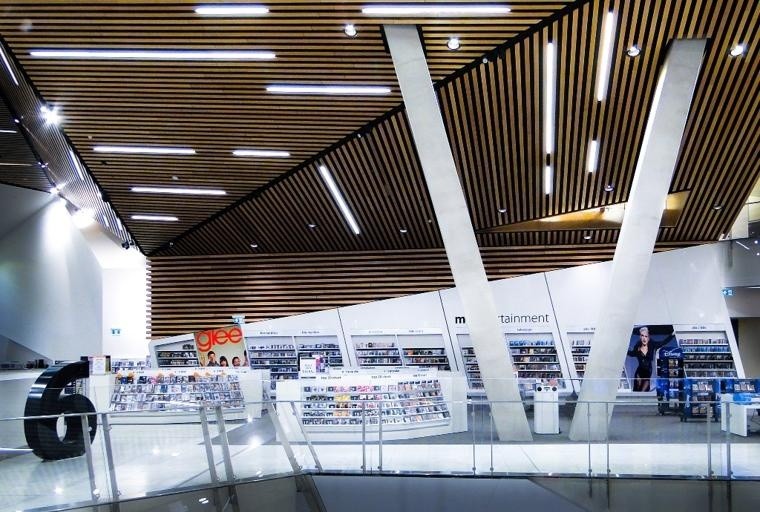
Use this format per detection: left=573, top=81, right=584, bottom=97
left=656, top=330, right=760, bottom=436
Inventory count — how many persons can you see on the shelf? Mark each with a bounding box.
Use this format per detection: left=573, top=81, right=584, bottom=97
left=205, top=350, right=219, bottom=367
left=232, top=356, right=241, bottom=367
left=219, top=356, right=230, bottom=367
left=243, top=350, right=249, bottom=367
left=627, top=325, right=675, bottom=392
